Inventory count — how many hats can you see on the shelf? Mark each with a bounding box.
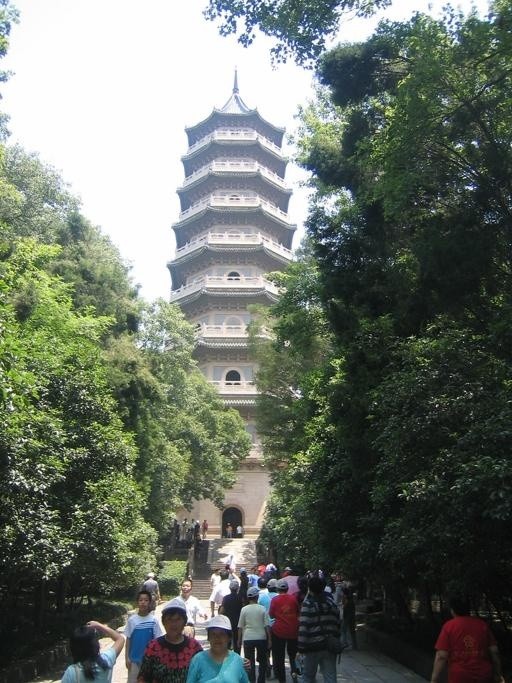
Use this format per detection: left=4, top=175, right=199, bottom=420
left=266, top=578, right=278, bottom=588
left=228, top=580, right=240, bottom=589
left=246, top=586, right=261, bottom=598
left=239, top=566, right=247, bottom=574
left=265, top=562, right=278, bottom=572
left=283, top=565, right=292, bottom=571
left=275, top=578, right=289, bottom=590
left=201, top=612, right=235, bottom=633
left=160, top=596, right=188, bottom=614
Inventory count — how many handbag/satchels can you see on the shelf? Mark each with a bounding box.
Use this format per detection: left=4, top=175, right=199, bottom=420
left=324, top=632, right=349, bottom=655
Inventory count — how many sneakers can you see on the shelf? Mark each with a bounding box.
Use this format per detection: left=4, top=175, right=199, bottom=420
left=266, top=667, right=305, bottom=683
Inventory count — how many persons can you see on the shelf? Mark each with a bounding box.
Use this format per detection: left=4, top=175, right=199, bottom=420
left=172, top=516, right=207, bottom=546
left=224, top=521, right=243, bottom=540
left=59, top=561, right=359, bottom=683
left=430, top=597, right=505, bottom=683
